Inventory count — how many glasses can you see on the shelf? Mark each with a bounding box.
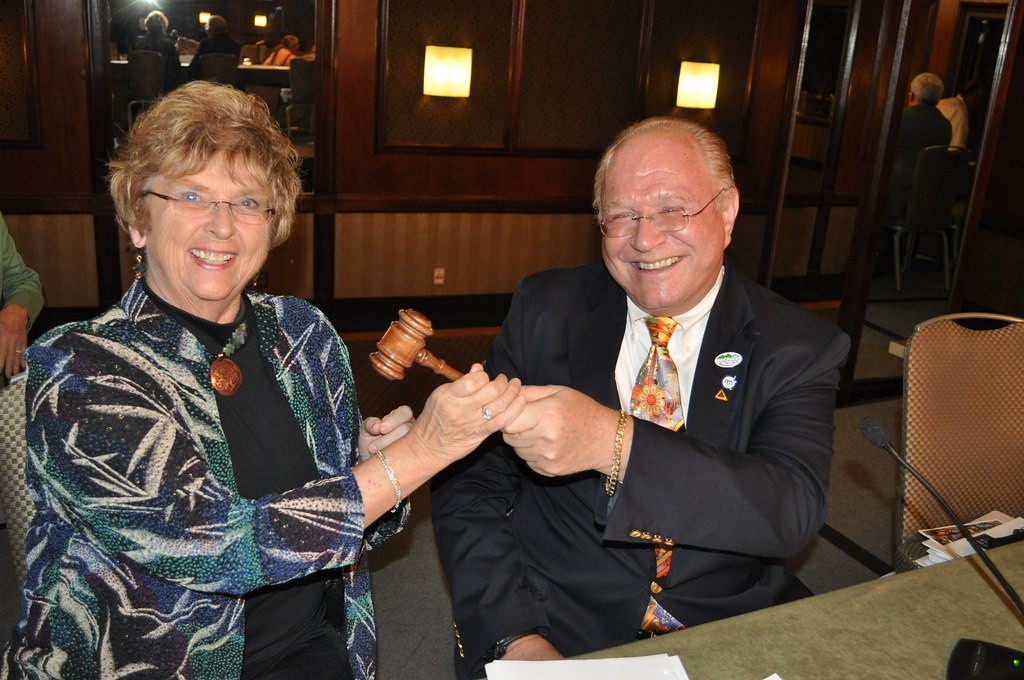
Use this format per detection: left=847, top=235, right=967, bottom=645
left=141, top=189, right=276, bottom=226
left=599, top=189, right=725, bottom=238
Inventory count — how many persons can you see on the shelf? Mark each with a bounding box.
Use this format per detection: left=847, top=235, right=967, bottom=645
left=111, top=8, right=236, bottom=78
left=883, top=74, right=952, bottom=160
left=936, top=81, right=981, bottom=150
left=0, top=214, right=44, bottom=378
left=0, top=82, right=526, bottom=680
left=432, top=118, right=852, bottom=680
left=262, top=34, right=297, bottom=69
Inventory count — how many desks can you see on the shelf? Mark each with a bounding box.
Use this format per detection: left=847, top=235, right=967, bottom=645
left=111, top=60, right=289, bottom=89
left=565, top=540, right=1024, bottom=680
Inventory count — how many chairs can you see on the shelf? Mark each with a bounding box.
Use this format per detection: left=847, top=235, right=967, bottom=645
left=126, top=50, right=165, bottom=136
left=200, top=55, right=234, bottom=87
left=891, top=313, right=1023, bottom=573
left=878, top=146, right=976, bottom=292
left=286, top=56, right=316, bottom=139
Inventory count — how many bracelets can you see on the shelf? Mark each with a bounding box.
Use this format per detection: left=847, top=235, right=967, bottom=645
left=489, top=629, right=535, bottom=660
left=605, top=407, right=627, bottom=496
left=376, top=451, right=402, bottom=512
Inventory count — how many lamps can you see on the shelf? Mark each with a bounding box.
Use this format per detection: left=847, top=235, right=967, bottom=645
left=676, top=62, right=721, bottom=110
left=425, top=47, right=473, bottom=98
left=198, top=12, right=211, bottom=23
left=254, top=15, right=267, bottom=27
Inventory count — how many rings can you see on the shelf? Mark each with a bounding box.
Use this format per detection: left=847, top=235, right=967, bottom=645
left=481, top=405, right=494, bottom=420
left=15, top=349, right=22, bottom=353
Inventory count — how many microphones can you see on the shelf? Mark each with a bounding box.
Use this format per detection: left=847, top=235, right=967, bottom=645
left=857, top=416, right=1024, bottom=618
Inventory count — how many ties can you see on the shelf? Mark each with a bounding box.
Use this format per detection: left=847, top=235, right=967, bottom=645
left=628, top=315, right=686, bottom=634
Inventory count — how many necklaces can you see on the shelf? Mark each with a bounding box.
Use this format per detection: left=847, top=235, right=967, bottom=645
left=173, top=318, right=249, bottom=396
